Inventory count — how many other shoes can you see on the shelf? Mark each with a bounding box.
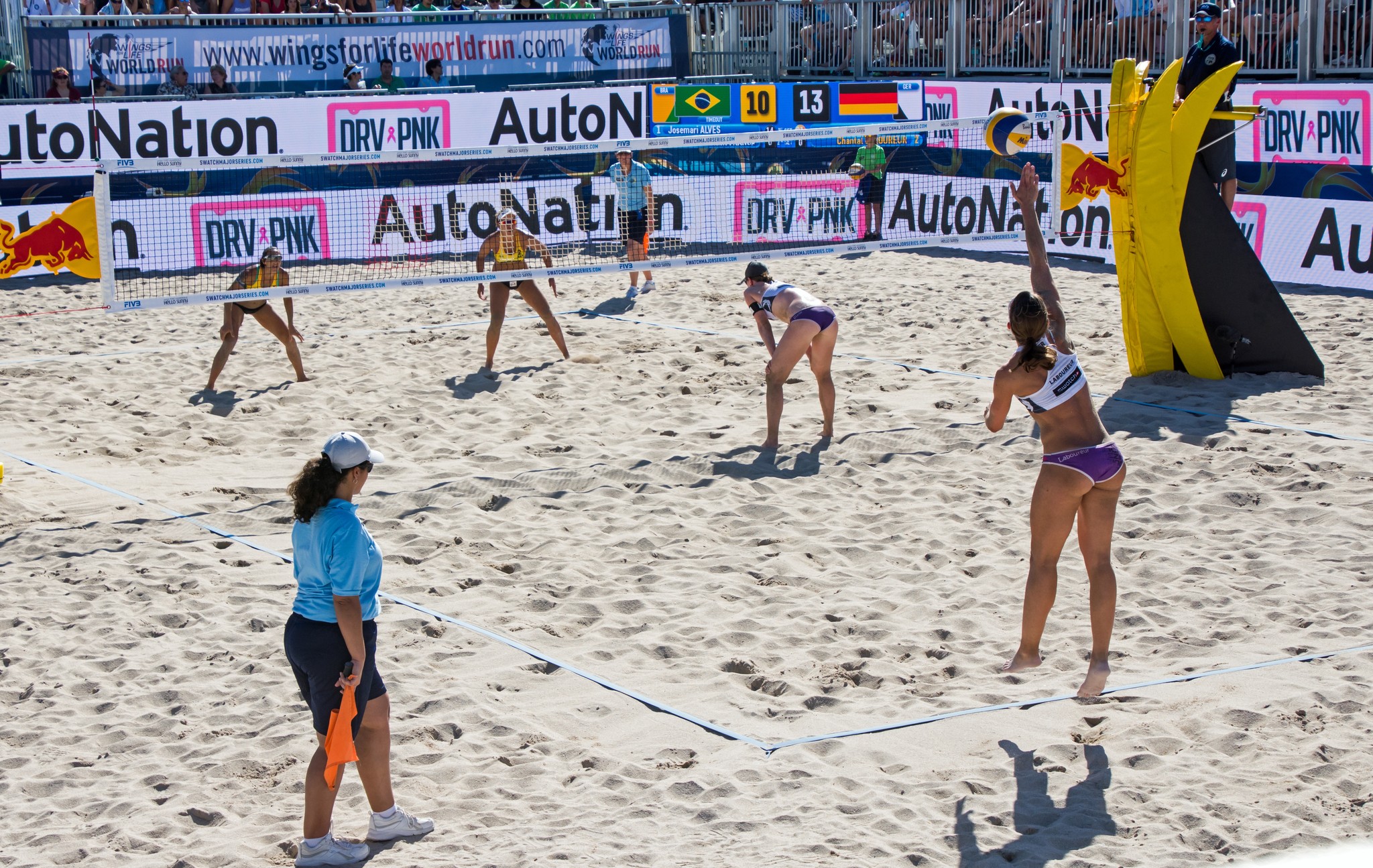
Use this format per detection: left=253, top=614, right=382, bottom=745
left=1088, top=60, right=1112, bottom=68
left=862, top=229, right=881, bottom=242
left=1024, top=61, right=1044, bottom=67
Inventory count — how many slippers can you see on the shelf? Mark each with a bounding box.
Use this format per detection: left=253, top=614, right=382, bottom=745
left=983, top=52, right=1004, bottom=58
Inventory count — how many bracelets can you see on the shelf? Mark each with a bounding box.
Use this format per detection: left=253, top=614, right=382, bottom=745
left=237, top=275, right=246, bottom=287
left=748, top=302, right=765, bottom=315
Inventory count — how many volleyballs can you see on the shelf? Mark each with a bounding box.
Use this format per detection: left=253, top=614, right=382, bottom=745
left=983, top=107, right=1032, bottom=157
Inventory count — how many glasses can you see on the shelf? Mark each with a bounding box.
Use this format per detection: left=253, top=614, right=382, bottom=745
left=618, top=153, right=631, bottom=156
left=490, top=0, right=500, bottom=3
left=436, top=64, right=442, bottom=67
left=356, top=463, right=374, bottom=473
left=263, top=255, right=283, bottom=261
left=1195, top=16, right=1212, bottom=22
left=180, top=0, right=189, bottom=2
left=499, top=219, right=517, bottom=225
left=863, top=135, right=871, bottom=137
left=177, top=72, right=188, bottom=75
left=111, top=0, right=122, bottom=4
left=56, top=76, right=67, bottom=79
left=353, top=71, right=360, bottom=74
left=99, top=83, right=106, bottom=88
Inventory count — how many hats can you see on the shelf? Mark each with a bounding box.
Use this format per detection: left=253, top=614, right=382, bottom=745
left=616, top=150, right=631, bottom=154
left=1193, top=2, right=1221, bottom=18
left=498, top=210, right=517, bottom=221
left=55, top=70, right=68, bottom=76
left=737, top=260, right=769, bottom=285
left=347, top=65, right=364, bottom=76
left=320, top=431, right=384, bottom=474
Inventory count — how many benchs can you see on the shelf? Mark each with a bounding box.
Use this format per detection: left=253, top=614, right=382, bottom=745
left=696, top=31, right=1366, bottom=80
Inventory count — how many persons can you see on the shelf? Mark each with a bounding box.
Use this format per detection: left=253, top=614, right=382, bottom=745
left=157, top=64, right=199, bottom=100
left=204, top=246, right=309, bottom=392
left=734, top=261, right=838, bottom=452
left=203, top=63, right=243, bottom=99
left=86, top=75, right=127, bottom=103
left=853, top=134, right=887, bottom=241
left=608, top=149, right=654, bottom=300
left=476, top=207, right=569, bottom=368
left=1172, top=1, right=1242, bottom=212
left=81, top=0, right=681, bottom=28
left=415, top=58, right=453, bottom=94
left=799, top=0, right=1373, bottom=76
left=340, top=64, right=382, bottom=91
left=45, top=67, right=81, bottom=104
left=0, top=58, right=15, bottom=79
left=983, top=161, right=1125, bottom=698
left=282, top=427, right=435, bottom=868
left=371, top=58, right=409, bottom=94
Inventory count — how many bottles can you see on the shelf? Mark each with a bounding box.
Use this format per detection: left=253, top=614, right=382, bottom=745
left=900, top=12, right=904, bottom=19
left=872, top=72, right=884, bottom=77
left=912, top=73, right=923, bottom=77
left=800, top=58, right=808, bottom=75
left=900, top=66, right=904, bottom=77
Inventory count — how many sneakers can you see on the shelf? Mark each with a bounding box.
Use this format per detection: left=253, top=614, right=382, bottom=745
left=1329, top=54, right=1355, bottom=65
left=294, top=832, right=370, bottom=867
left=367, top=807, right=435, bottom=840
left=637, top=281, right=655, bottom=292
left=625, top=288, right=638, bottom=297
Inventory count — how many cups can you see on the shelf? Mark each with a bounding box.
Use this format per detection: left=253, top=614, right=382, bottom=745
left=357, top=81, right=366, bottom=90
